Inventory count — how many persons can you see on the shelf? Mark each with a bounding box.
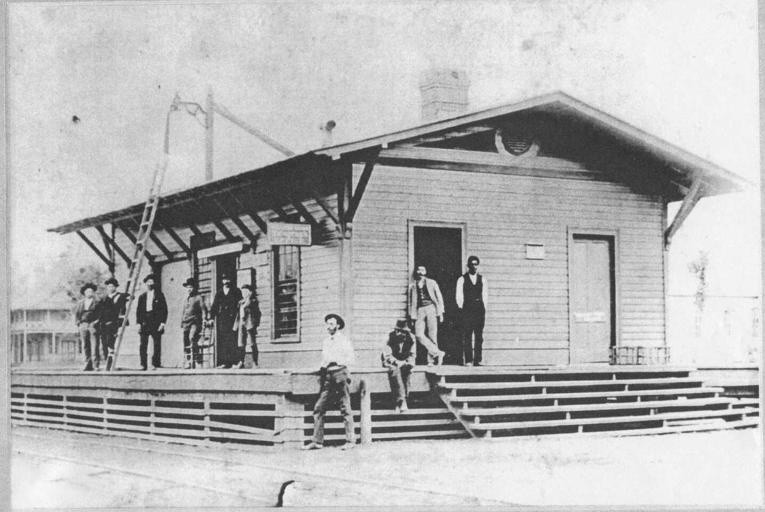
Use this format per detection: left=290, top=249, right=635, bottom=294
left=407, top=263, right=447, bottom=368
left=177, top=278, right=204, bottom=367
left=72, top=279, right=102, bottom=371
left=232, top=284, right=261, bottom=368
left=207, top=273, right=237, bottom=370
left=297, top=313, right=355, bottom=451
left=134, top=273, right=170, bottom=371
left=382, top=319, right=417, bottom=413
left=454, top=255, right=486, bottom=366
left=94, top=277, right=129, bottom=364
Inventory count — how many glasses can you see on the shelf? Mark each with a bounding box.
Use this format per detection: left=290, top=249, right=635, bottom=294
left=470, top=263, right=477, bottom=267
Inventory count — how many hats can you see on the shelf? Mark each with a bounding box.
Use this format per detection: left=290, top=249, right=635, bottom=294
left=325, top=314, right=344, bottom=330
left=143, top=274, right=158, bottom=282
left=220, top=273, right=233, bottom=279
left=80, top=283, right=96, bottom=294
left=182, top=278, right=197, bottom=287
left=393, top=319, right=410, bottom=331
left=104, top=278, right=118, bottom=287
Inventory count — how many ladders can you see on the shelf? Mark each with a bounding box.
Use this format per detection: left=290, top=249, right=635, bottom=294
left=104, top=150, right=171, bottom=372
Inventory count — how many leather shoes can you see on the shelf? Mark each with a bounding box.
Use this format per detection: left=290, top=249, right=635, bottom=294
left=465, top=363, right=472, bottom=366
left=137, top=366, right=146, bottom=371
left=151, top=366, right=163, bottom=371
left=236, top=362, right=244, bottom=369
left=341, top=442, right=356, bottom=451
left=303, top=443, right=323, bottom=451
left=399, top=404, right=407, bottom=412
left=474, top=362, right=484, bottom=366
left=437, top=351, right=445, bottom=365
